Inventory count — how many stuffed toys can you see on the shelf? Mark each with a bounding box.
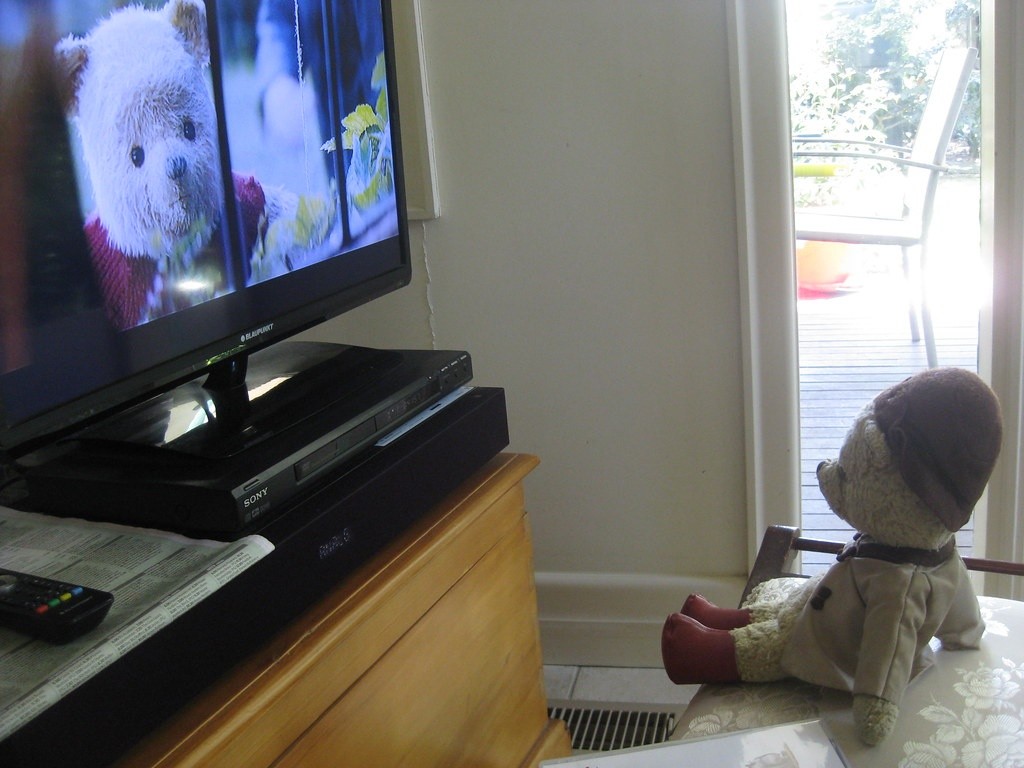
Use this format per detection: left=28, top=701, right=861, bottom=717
left=661, top=368, right=1003, bottom=746
left=49, top=0, right=299, bottom=331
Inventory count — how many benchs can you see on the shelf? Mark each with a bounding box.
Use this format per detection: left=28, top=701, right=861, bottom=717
left=667, top=524, right=1024, bottom=768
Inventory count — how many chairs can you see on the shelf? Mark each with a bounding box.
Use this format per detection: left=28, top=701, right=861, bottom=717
left=791, top=48, right=978, bottom=370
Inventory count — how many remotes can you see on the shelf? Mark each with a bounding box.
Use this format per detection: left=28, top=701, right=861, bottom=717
left=0, top=567, right=114, bottom=645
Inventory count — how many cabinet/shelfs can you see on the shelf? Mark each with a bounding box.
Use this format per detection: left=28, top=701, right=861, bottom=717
left=111, top=441, right=574, bottom=768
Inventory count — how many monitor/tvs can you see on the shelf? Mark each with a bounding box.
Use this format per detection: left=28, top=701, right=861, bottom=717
left=0, top=0, right=412, bottom=461
